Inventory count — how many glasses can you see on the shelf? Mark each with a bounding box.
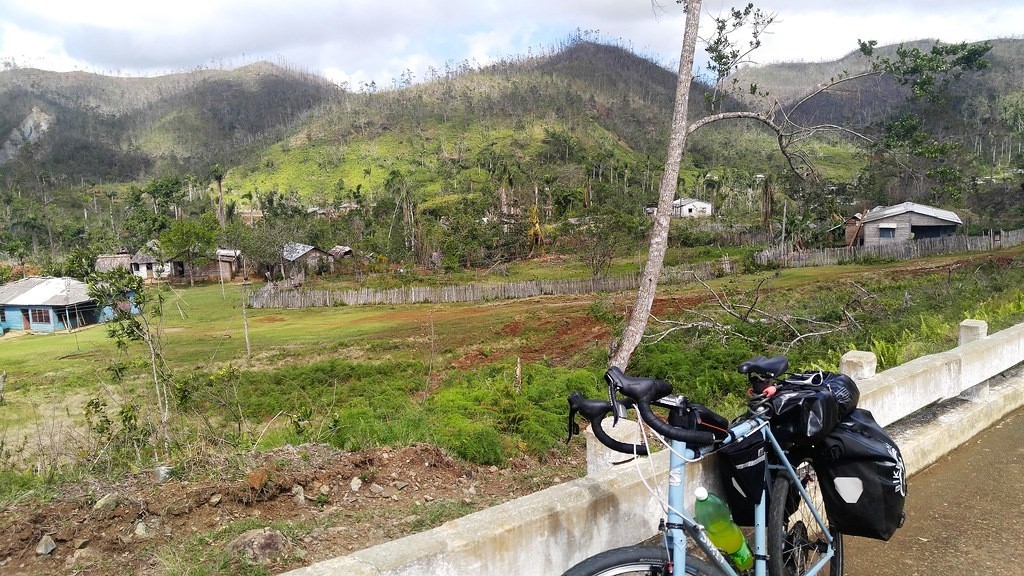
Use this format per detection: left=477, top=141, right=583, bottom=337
left=783, top=369, right=824, bottom=387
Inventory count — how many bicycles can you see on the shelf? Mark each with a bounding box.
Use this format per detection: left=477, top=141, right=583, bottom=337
left=557, top=353, right=848, bottom=576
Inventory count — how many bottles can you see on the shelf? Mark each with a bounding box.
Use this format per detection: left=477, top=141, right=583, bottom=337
left=693, top=485, right=754, bottom=572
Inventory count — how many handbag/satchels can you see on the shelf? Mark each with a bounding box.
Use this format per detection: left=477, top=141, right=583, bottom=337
left=715, top=408, right=801, bottom=527
left=812, top=409, right=906, bottom=541
left=767, top=371, right=859, bottom=459
